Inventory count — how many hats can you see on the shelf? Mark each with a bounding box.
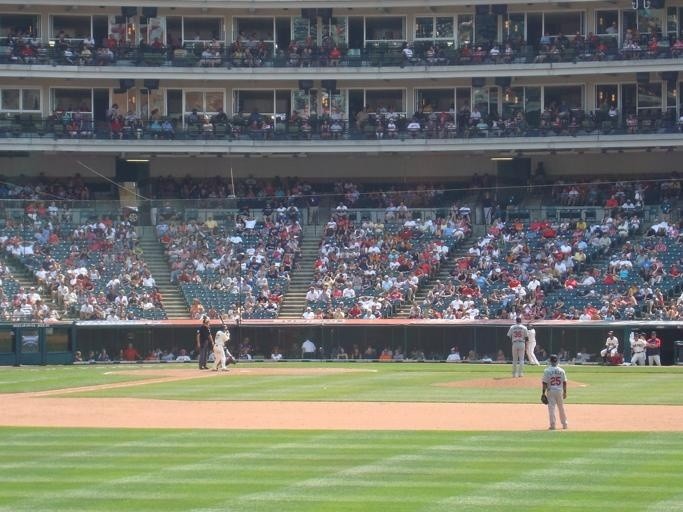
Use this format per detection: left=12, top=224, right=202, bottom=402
left=517, top=318, right=521, bottom=322
left=551, top=355, right=557, bottom=362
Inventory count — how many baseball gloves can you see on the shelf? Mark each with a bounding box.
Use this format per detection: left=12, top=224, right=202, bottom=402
left=541, top=394, right=549, bottom=405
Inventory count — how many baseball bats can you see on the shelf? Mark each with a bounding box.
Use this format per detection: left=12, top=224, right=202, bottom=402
left=219, top=313, right=225, bottom=327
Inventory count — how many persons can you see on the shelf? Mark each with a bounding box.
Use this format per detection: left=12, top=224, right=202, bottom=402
left=542, top=356, right=568, bottom=429
